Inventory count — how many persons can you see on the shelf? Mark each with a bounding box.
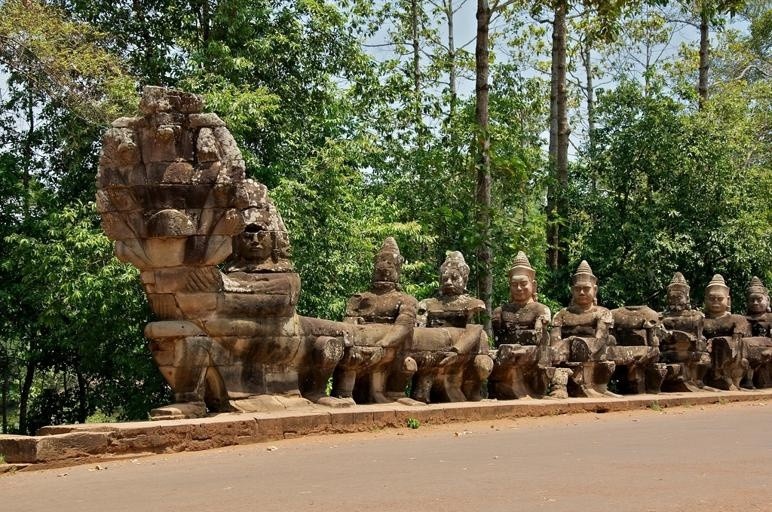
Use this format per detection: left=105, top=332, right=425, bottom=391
left=659, top=272, right=710, bottom=392
left=418, top=251, right=494, bottom=398
left=337, top=235, right=418, bottom=399
left=492, top=251, right=551, bottom=399
left=227, top=177, right=294, bottom=273
left=611, top=305, right=661, bottom=394
left=747, top=276, right=771, bottom=388
left=551, top=260, right=614, bottom=399
left=702, top=274, right=747, bottom=390
